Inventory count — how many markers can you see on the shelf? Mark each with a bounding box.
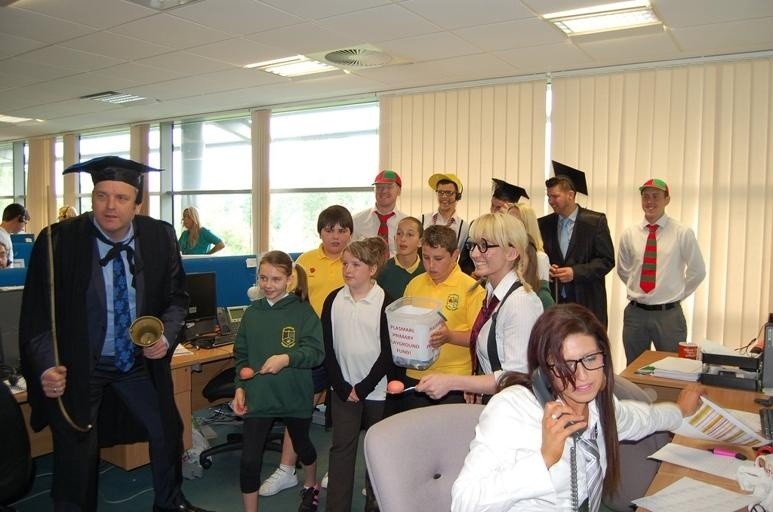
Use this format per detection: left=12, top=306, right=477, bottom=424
left=709, top=448, right=747, bottom=460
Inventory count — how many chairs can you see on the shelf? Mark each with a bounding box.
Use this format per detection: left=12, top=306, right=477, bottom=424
left=596, top=375, right=670, bottom=511
left=198, top=364, right=329, bottom=470
left=362, top=403, right=486, bottom=510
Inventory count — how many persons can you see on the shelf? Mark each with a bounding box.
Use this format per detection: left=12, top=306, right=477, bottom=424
left=178, top=207, right=225, bottom=255
left=18, top=155, right=207, bottom=510
left=231, top=250, right=325, bottom=511
left=321, top=217, right=426, bottom=495
left=320, top=237, right=391, bottom=512
left=399, top=224, right=486, bottom=411
left=609, top=177, right=708, bottom=365
left=58, top=207, right=77, bottom=222
left=258, top=205, right=354, bottom=497
left=353, top=166, right=555, bottom=309
left=0, top=203, right=30, bottom=268
left=447, top=297, right=713, bottom=511
left=538, top=159, right=615, bottom=336
left=415, top=214, right=543, bottom=405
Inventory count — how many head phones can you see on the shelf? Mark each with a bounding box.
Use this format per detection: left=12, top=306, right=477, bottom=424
left=63, top=206, right=69, bottom=217
left=0, top=242, right=12, bottom=267
left=19, top=208, right=26, bottom=222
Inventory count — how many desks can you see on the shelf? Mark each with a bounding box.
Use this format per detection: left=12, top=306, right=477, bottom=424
left=96, top=341, right=257, bottom=474
left=618, top=348, right=772, bottom=511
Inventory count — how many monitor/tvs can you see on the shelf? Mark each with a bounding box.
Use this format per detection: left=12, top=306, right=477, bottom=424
left=184, top=271, right=217, bottom=323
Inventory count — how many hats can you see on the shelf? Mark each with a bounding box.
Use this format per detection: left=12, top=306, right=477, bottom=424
left=63, top=155, right=166, bottom=206
left=640, top=179, right=670, bottom=193
left=372, top=170, right=402, bottom=188
left=492, top=178, right=529, bottom=203
left=546, top=160, right=589, bottom=196
left=428, top=173, right=463, bottom=193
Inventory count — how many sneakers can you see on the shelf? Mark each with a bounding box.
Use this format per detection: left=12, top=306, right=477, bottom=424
left=258, top=466, right=299, bottom=496
left=299, top=483, right=321, bottom=512
left=321, top=472, right=329, bottom=488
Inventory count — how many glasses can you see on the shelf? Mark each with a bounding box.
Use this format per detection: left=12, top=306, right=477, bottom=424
left=436, top=189, right=456, bottom=197
left=546, top=351, right=605, bottom=378
left=464, top=238, right=499, bottom=253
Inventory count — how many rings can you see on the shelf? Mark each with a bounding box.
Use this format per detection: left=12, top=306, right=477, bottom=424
left=550, top=413, right=557, bottom=420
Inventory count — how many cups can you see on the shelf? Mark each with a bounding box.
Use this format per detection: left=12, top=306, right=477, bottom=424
left=128, top=315, right=164, bottom=347
left=678, top=342, right=697, bottom=360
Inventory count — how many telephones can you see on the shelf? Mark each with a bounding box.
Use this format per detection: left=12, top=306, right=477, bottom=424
left=531, top=365, right=587, bottom=438
left=217, top=305, right=253, bottom=336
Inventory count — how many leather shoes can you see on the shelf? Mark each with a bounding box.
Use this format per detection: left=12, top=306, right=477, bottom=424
left=174, top=501, right=210, bottom=512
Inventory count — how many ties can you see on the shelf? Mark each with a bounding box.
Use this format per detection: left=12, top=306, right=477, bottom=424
left=560, top=219, right=569, bottom=298
left=576, top=424, right=604, bottom=511
left=469, top=291, right=500, bottom=373
left=639, top=225, right=659, bottom=294
left=375, top=211, right=395, bottom=260
left=114, top=256, right=136, bottom=373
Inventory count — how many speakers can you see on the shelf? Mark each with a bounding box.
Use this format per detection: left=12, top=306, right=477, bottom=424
left=217, top=307, right=233, bottom=335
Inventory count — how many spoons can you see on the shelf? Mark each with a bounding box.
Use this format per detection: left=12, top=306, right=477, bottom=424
left=239, top=371, right=259, bottom=380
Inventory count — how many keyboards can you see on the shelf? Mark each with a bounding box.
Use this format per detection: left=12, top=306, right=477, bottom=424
left=759, top=408, right=773, bottom=440
left=212, top=335, right=234, bottom=349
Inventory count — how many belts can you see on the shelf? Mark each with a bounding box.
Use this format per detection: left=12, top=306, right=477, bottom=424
left=630, top=299, right=676, bottom=310
